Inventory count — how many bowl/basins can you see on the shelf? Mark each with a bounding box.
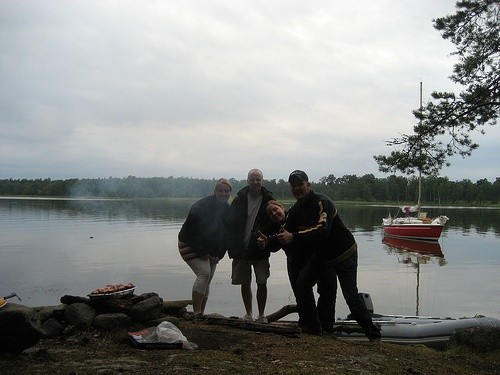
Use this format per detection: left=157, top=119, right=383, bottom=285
left=86, top=285, right=137, bottom=300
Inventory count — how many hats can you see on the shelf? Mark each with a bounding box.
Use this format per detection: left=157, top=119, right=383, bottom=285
left=289, top=170, right=309, bottom=181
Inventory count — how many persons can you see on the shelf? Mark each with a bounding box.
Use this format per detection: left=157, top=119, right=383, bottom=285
left=257, top=170, right=381, bottom=342
left=179, top=180, right=231, bottom=322
left=227, top=171, right=277, bottom=323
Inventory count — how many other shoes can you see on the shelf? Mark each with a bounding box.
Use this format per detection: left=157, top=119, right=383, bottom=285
left=245, top=315, right=253, bottom=324
left=192, top=313, right=208, bottom=321
left=258, top=316, right=268, bottom=324
left=370, top=325, right=381, bottom=342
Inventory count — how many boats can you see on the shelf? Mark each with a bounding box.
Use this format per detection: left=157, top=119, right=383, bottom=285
left=382, top=235, right=444, bottom=257
left=383, top=224, right=445, bottom=240
left=333, top=293, right=500, bottom=337
